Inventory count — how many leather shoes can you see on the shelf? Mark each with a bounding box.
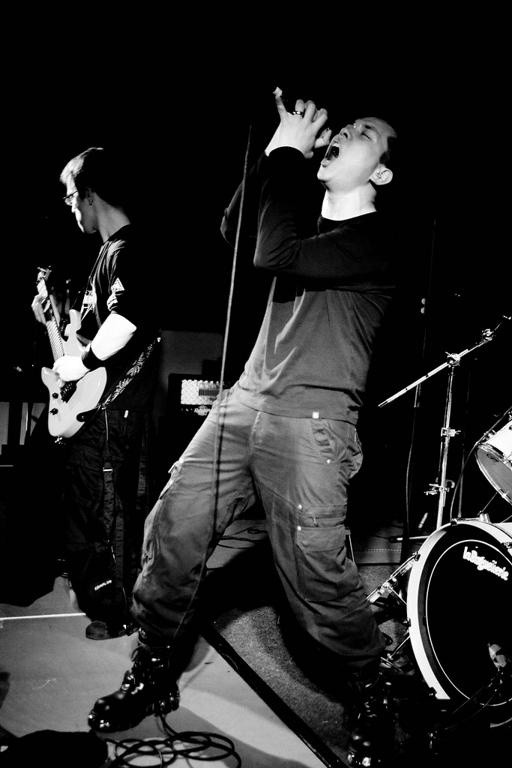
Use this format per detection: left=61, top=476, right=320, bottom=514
left=86, top=618, right=133, bottom=640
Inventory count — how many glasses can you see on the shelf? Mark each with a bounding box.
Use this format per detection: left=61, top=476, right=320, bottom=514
left=62, top=190, right=79, bottom=206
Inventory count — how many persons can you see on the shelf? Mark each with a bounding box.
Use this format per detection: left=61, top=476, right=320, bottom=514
left=88, top=98, right=416, bottom=732
left=32, top=145, right=163, bottom=640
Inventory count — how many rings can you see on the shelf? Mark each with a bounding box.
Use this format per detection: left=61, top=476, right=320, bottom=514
left=294, top=111, right=305, bottom=116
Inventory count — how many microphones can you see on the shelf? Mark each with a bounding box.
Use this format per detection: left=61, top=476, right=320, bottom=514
left=273, top=85, right=330, bottom=139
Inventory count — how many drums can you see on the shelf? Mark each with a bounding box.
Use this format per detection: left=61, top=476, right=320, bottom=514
left=474, top=402, right=512, bottom=505
left=404, top=511, right=512, bottom=732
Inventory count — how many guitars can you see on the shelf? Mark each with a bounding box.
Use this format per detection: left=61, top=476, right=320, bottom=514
left=36, top=265, right=107, bottom=439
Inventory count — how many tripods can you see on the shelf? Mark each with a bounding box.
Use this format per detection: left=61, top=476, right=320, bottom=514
left=365, top=319, right=502, bottom=638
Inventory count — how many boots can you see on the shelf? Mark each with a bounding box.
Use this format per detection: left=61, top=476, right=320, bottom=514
left=344, top=659, right=393, bottom=768
left=87, top=630, right=180, bottom=734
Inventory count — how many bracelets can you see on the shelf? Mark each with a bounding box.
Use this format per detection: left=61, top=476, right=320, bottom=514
left=83, top=347, right=104, bottom=370
